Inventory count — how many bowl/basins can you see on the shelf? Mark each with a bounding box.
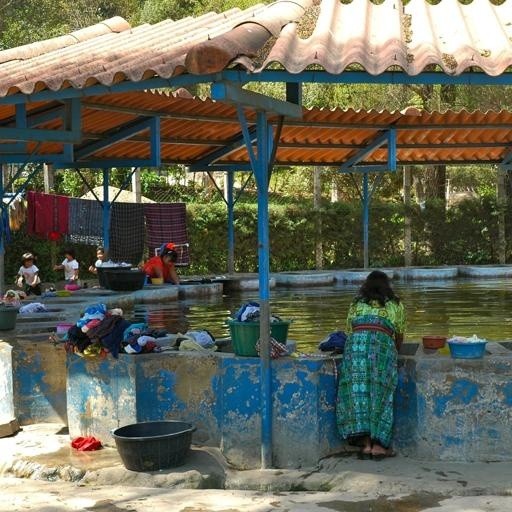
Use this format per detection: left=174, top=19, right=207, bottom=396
left=423, top=334, right=488, bottom=360
left=151, top=278, right=163, bottom=285
left=95, top=264, right=146, bottom=291
left=224, top=319, right=293, bottom=357
left=111, top=420, right=196, bottom=470
left=0, top=305, right=19, bottom=332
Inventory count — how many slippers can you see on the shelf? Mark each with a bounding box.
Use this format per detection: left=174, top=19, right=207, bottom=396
left=360, top=447, right=396, bottom=461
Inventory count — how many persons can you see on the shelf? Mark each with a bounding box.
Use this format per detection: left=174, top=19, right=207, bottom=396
left=14, top=253, right=42, bottom=296
left=140, top=248, right=180, bottom=285
left=53, top=246, right=79, bottom=284
left=89, top=247, right=104, bottom=275
left=335, top=270, right=406, bottom=461
